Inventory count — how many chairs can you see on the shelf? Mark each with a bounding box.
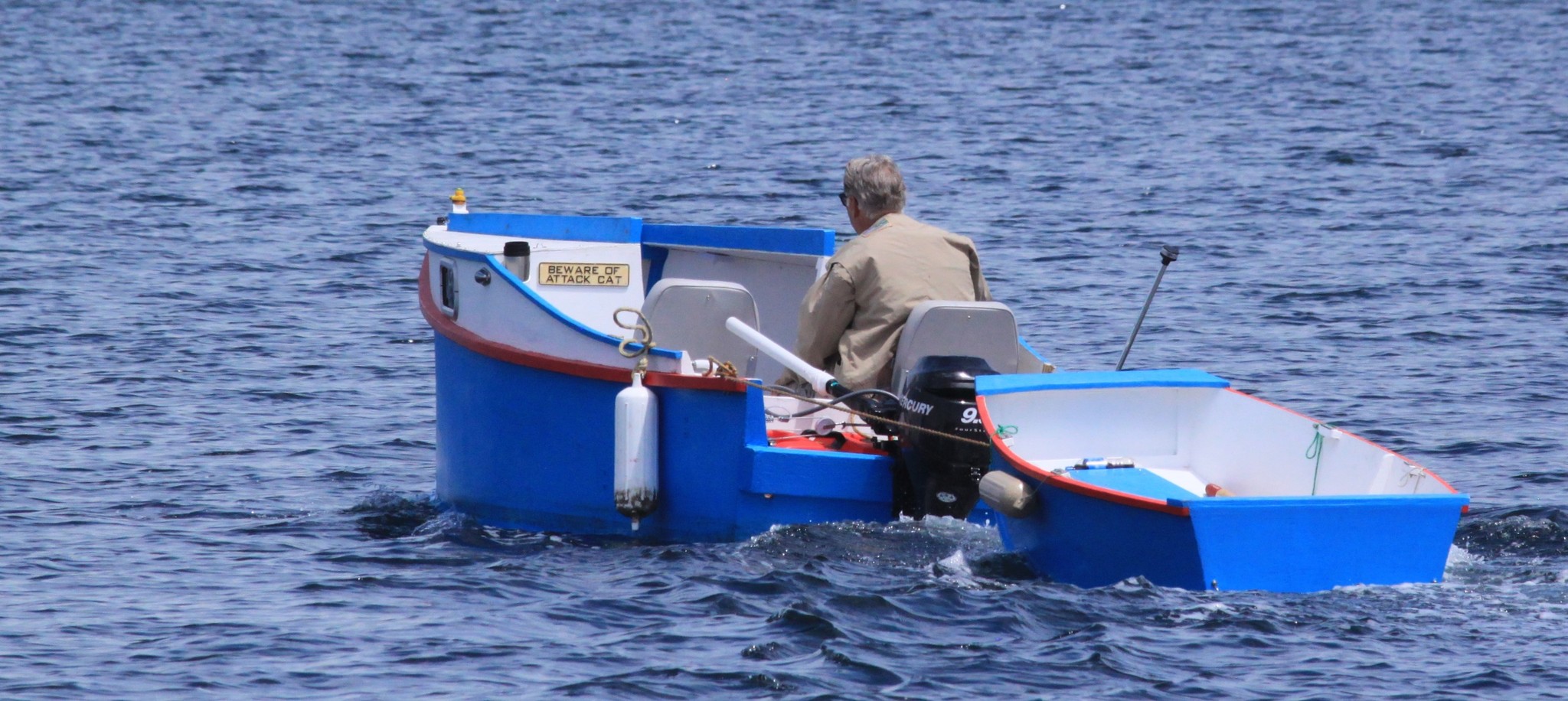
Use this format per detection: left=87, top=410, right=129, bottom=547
left=633, top=278, right=760, bottom=378
left=893, top=300, right=1019, bottom=397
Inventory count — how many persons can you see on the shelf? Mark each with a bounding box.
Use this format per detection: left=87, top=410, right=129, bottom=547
left=770, top=154, right=993, bottom=401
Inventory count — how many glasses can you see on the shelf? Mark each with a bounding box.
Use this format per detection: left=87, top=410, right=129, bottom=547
left=839, top=192, right=853, bottom=207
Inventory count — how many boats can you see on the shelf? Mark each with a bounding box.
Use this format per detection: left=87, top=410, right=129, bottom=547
left=960, top=367, right=1467, bottom=602
left=413, top=190, right=1077, bottom=547
